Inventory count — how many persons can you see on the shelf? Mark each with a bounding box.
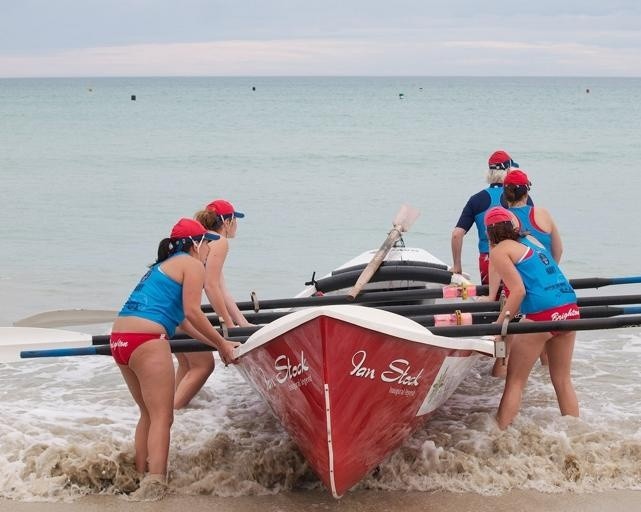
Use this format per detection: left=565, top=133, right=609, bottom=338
left=484, top=207, right=579, bottom=430
left=450, top=150, right=534, bottom=301
left=491, top=169, right=563, bottom=378
left=108, top=218, right=242, bottom=486
left=164, top=199, right=255, bottom=410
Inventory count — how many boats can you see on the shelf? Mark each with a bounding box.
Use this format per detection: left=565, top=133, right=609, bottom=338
left=232, top=247, right=495, bottom=499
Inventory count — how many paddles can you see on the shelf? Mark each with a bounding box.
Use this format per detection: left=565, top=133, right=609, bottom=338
left=0, top=307, right=641, bottom=364
left=347, top=201, right=420, bottom=301
left=13, top=294, right=641, bottom=327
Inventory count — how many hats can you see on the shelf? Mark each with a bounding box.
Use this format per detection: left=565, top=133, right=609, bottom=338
left=206, top=200, right=245, bottom=220
left=170, top=219, right=220, bottom=247
left=484, top=150, right=532, bottom=227
